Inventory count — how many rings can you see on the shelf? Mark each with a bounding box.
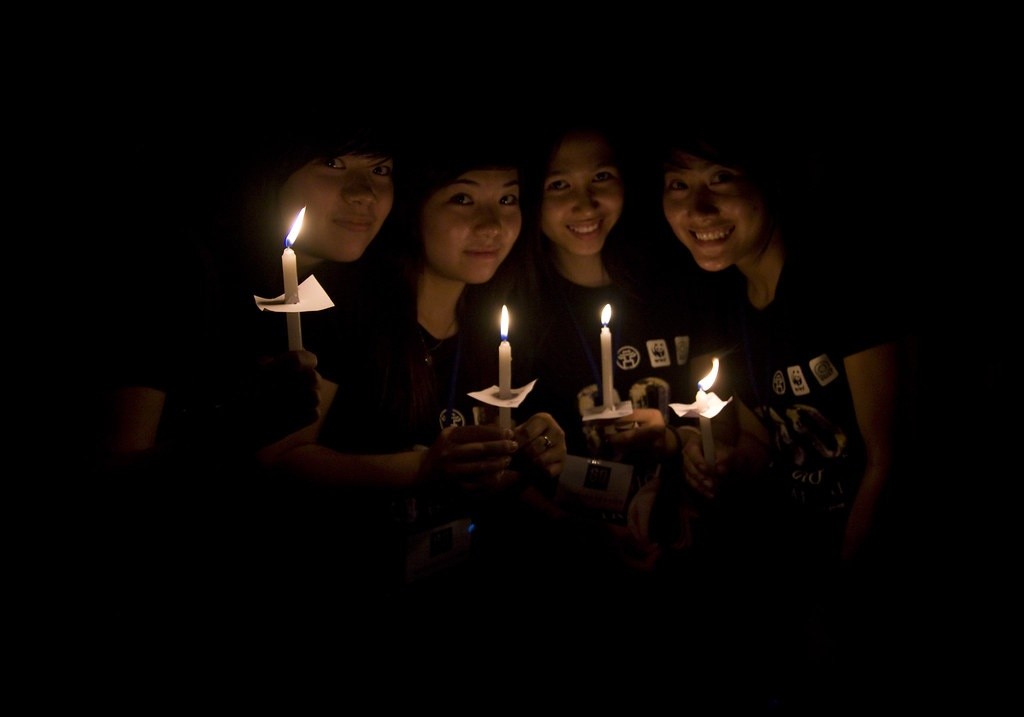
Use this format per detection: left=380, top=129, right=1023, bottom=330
left=543, top=433, right=554, bottom=449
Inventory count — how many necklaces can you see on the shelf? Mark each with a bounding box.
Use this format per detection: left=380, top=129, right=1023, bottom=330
left=418, top=319, right=456, bottom=363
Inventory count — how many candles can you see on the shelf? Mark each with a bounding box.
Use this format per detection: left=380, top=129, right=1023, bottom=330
left=498, top=304, right=512, bottom=430
left=601, top=303, right=615, bottom=434
left=281, top=204, right=307, bottom=352
left=695, top=357, right=719, bottom=459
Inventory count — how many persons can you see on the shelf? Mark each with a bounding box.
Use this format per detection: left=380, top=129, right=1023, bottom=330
left=110, top=91, right=909, bottom=631
